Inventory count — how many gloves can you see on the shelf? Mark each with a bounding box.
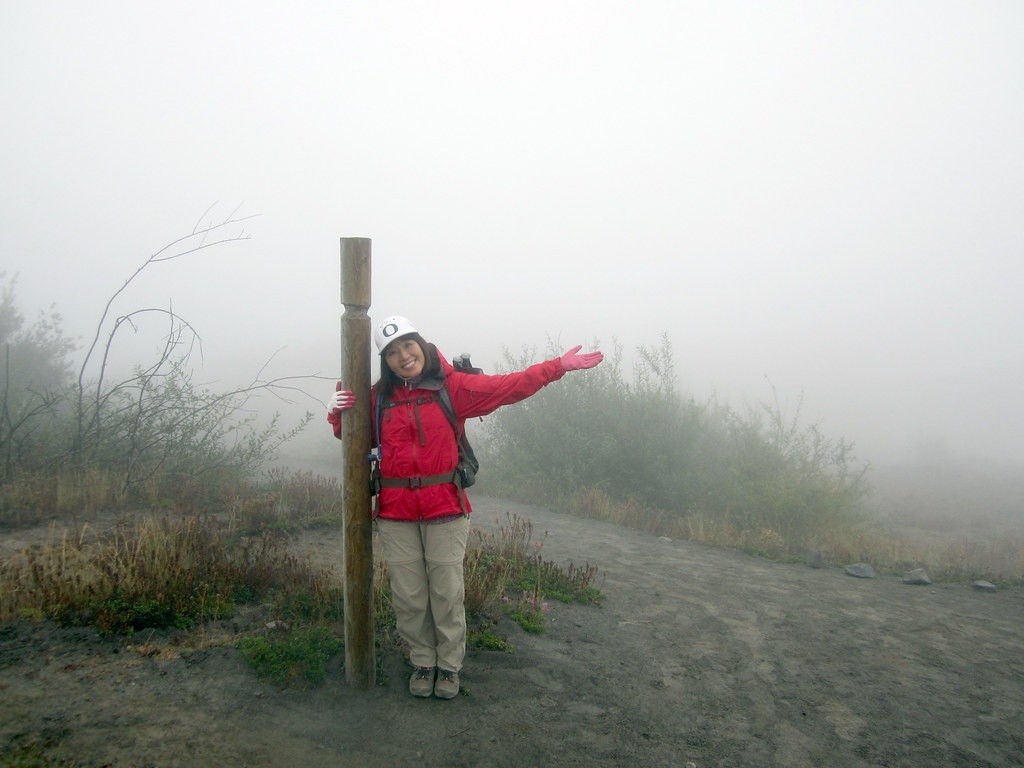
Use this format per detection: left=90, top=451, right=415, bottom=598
left=559, top=344, right=604, bottom=373
left=327, top=381, right=355, bottom=417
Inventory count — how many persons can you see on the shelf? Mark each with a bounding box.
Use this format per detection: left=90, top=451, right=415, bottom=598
left=328, top=316, right=603, bottom=702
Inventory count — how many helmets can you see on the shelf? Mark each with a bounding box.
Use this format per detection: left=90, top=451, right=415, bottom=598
left=373, top=316, right=419, bottom=356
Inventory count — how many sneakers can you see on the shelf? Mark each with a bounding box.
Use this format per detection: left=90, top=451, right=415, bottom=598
left=435, top=665, right=460, bottom=701
left=409, top=666, right=433, bottom=700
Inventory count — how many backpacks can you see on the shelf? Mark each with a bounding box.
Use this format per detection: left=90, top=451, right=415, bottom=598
left=370, top=379, right=480, bottom=496
